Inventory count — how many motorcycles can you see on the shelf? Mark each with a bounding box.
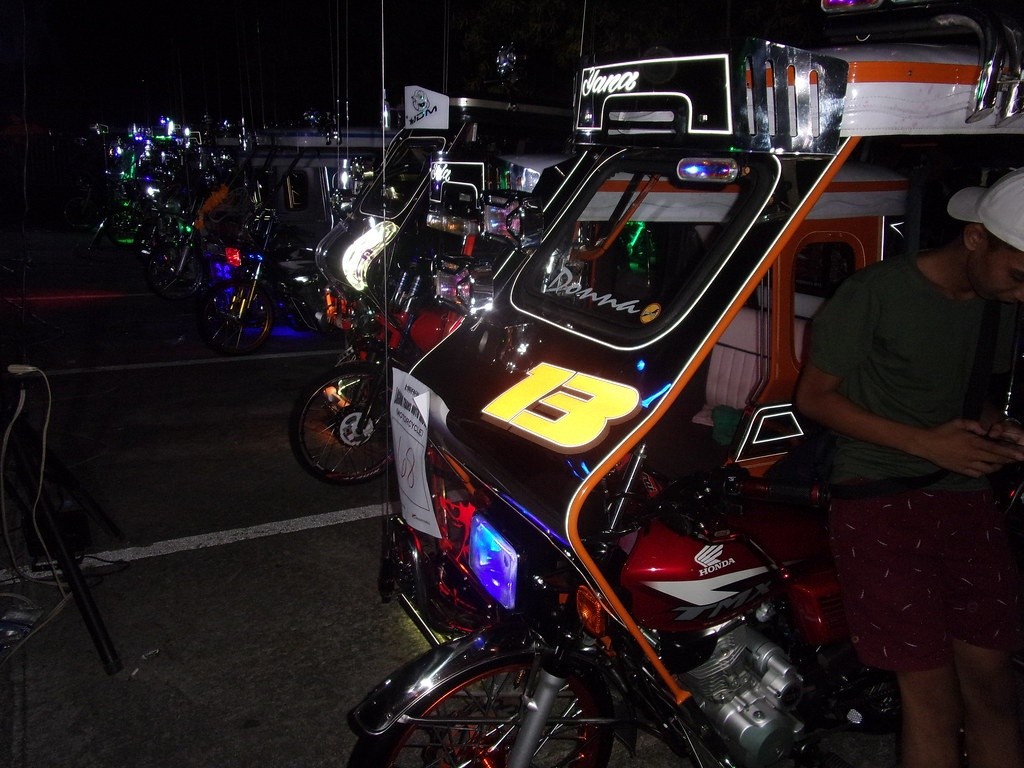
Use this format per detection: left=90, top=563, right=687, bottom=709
left=340, top=0, right=1024, bottom=768
left=89, top=85, right=577, bottom=487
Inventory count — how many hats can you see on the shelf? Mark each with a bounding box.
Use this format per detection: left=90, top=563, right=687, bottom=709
left=946, top=166, right=1024, bottom=253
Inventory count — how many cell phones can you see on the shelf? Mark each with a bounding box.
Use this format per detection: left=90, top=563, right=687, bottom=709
left=983, top=436, right=1024, bottom=455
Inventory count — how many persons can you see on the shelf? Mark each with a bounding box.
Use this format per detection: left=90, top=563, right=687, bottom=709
left=795, top=170, right=1024, bottom=768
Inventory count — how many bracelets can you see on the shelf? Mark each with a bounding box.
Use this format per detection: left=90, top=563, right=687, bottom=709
left=1004, top=417, right=1022, bottom=425
